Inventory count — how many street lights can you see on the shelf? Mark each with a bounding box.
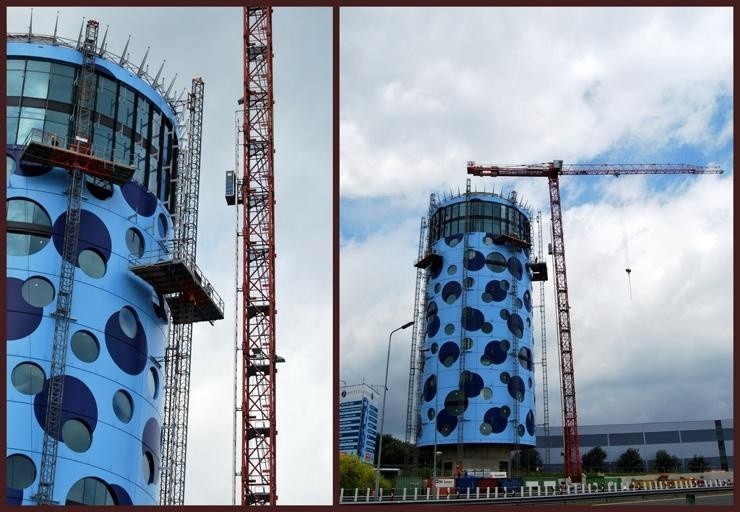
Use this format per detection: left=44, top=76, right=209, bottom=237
left=374, top=322, right=418, bottom=497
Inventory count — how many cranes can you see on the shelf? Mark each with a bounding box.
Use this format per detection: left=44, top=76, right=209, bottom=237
left=464, top=160, right=725, bottom=475
left=224, top=0, right=293, bottom=509
